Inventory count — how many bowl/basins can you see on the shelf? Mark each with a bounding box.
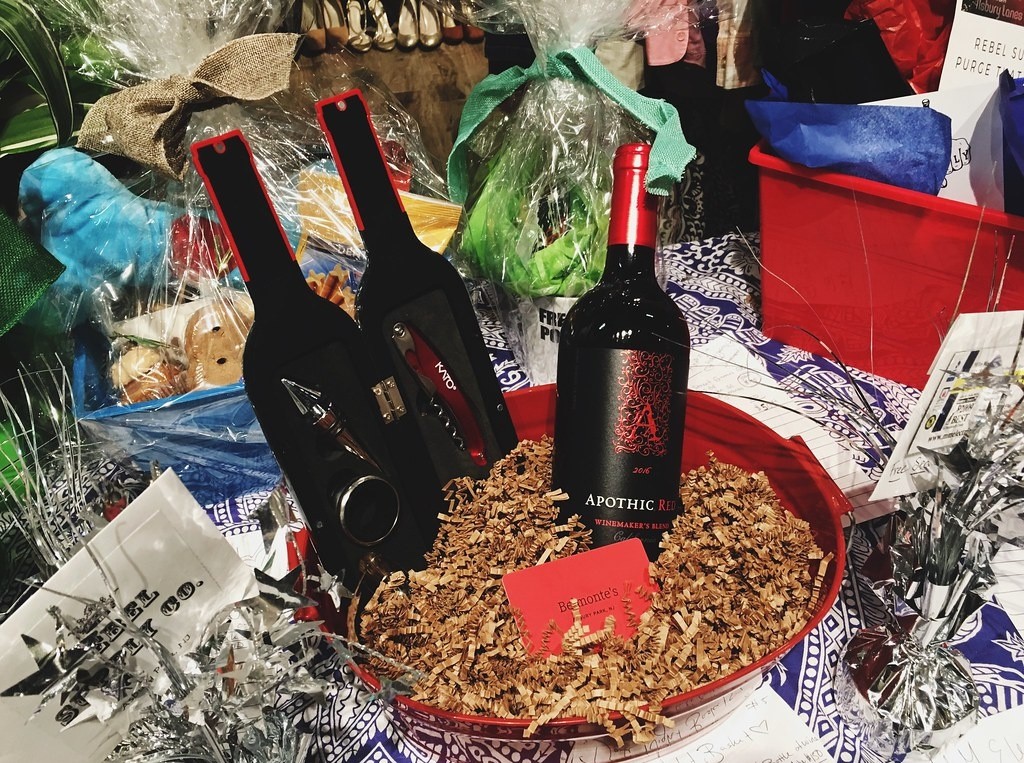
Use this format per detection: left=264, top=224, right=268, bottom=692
left=287, top=384, right=854, bottom=762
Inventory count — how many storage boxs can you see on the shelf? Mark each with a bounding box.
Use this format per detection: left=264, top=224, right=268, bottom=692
left=73, top=335, right=286, bottom=516
left=750, top=138, right=1024, bottom=391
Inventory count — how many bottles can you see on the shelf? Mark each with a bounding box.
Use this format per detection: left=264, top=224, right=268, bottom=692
left=315, top=88, right=526, bottom=502
left=191, top=129, right=448, bottom=607
left=551, top=143, right=691, bottom=563
